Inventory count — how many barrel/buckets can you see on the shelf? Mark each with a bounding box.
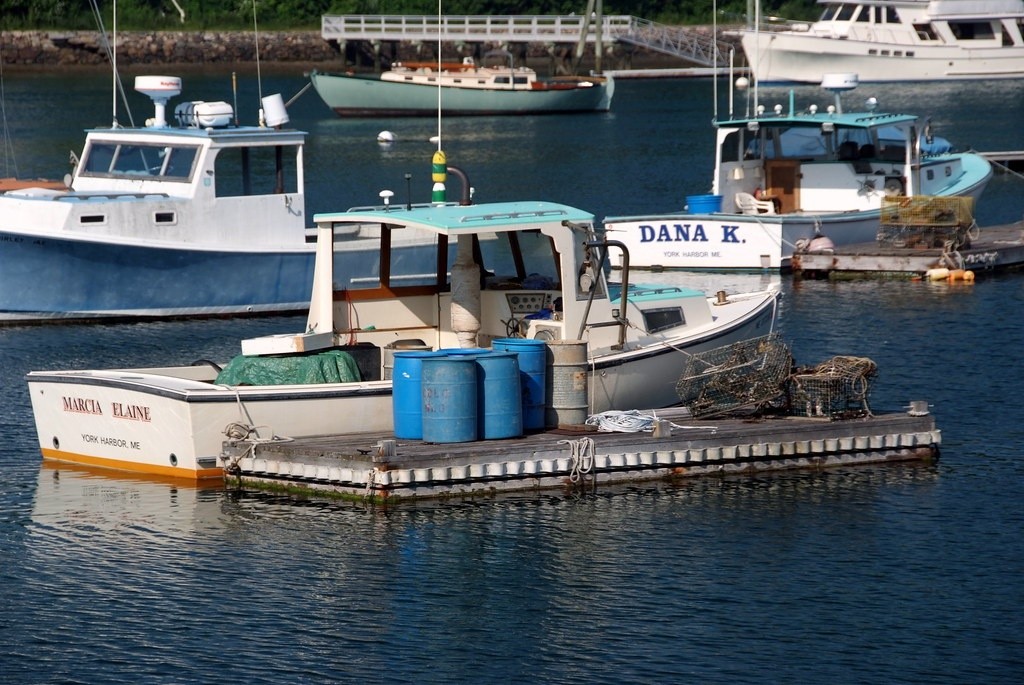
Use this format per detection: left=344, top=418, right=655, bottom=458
left=686, top=194, right=723, bottom=214
left=382, top=339, right=589, bottom=443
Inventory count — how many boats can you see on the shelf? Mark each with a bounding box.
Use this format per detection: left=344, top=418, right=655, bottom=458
left=0, top=63, right=500, bottom=323
left=741, top=0, right=1024, bottom=84
left=24, top=0, right=805, bottom=482
left=309, top=56, right=616, bottom=116
left=657, top=0, right=998, bottom=261
left=26, top=459, right=236, bottom=552
